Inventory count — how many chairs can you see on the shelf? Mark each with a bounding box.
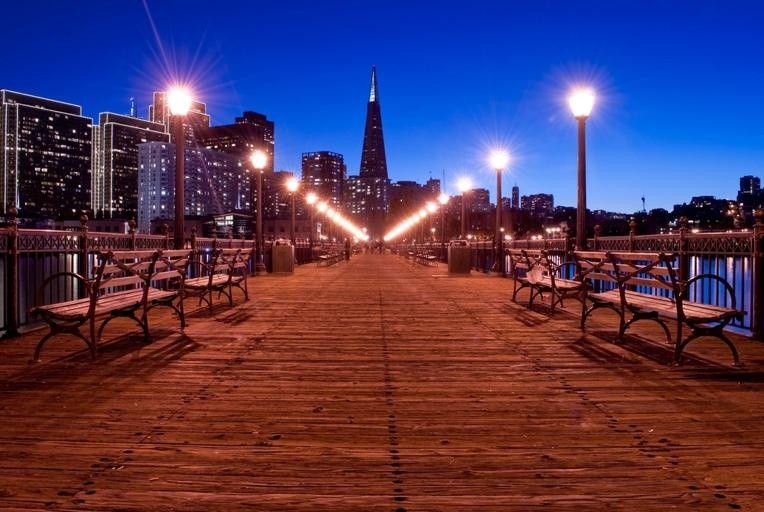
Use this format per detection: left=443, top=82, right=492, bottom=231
left=317, top=243, right=347, bottom=266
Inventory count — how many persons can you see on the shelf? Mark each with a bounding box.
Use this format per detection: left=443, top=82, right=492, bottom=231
left=344, top=237, right=384, bottom=260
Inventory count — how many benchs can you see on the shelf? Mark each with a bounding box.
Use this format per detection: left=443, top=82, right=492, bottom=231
left=412, top=247, right=438, bottom=266
left=503, top=248, right=593, bottom=313
left=568, top=249, right=747, bottom=370
left=26, top=247, right=194, bottom=361
left=175, top=249, right=255, bottom=317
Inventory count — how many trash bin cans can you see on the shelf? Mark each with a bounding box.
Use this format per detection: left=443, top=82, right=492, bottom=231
left=448, top=240, right=468, bottom=274
left=272, top=239, right=294, bottom=274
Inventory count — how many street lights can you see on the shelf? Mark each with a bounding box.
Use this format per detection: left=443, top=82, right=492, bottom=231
left=382, top=174, right=472, bottom=248
left=488, top=148, right=511, bottom=273
left=304, top=189, right=371, bottom=249
left=249, top=150, right=268, bottom=275
left=567, top=85, right=595, bottom=292
left=285, top=176, right=300, bottom=266
left=165, top=84, right=194, bottom=288
